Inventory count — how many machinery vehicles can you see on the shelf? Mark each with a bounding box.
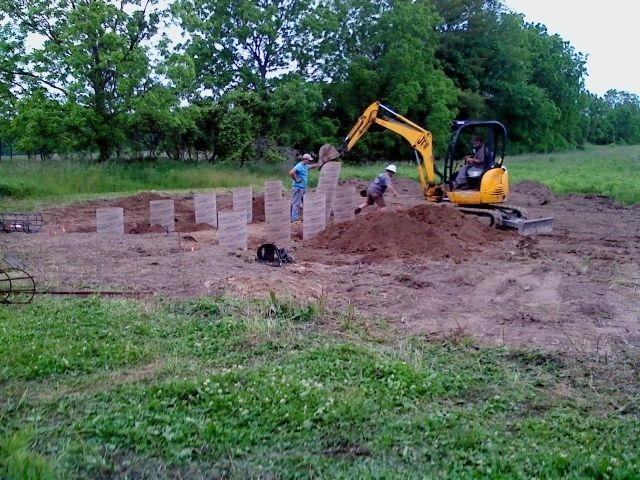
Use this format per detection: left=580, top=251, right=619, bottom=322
left=316, top=100, right=553, bottom=238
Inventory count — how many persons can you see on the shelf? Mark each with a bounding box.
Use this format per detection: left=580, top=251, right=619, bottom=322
left=353, top=165, right=397, bottom=215
left=288, top=154, right=318, bottom=223
left=452, top=135, right=492, bottom=188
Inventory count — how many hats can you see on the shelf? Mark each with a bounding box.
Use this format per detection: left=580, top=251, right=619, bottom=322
left=303, top=153, right=314, bottom=161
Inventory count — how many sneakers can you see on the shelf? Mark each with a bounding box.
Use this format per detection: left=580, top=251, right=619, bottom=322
left=354, top=207, right=361, bottom=214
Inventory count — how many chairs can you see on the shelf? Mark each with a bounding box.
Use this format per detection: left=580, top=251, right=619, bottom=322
left=467, top=141, right=496, bottom=177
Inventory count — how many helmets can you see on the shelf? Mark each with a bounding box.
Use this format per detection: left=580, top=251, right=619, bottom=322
left=386, top=165, right=397, bottom=173
